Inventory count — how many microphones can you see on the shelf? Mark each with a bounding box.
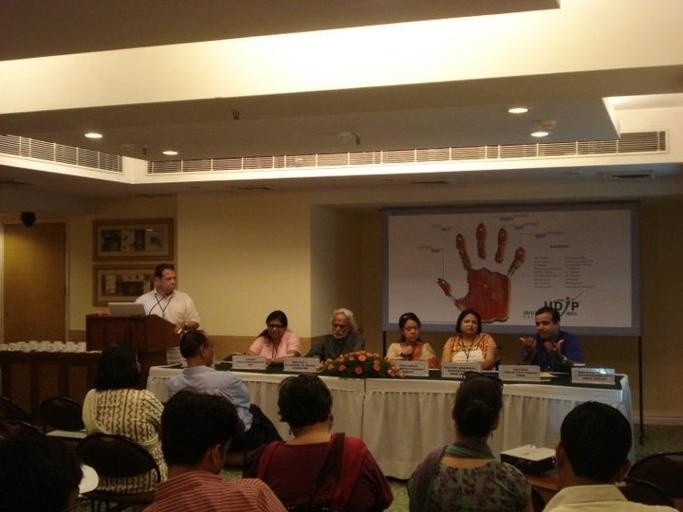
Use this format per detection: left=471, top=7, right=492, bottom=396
left=149, top=292, right=169, bottom=315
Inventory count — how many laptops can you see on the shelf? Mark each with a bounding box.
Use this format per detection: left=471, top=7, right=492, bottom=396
left=107, top=302, right=146, bottom=317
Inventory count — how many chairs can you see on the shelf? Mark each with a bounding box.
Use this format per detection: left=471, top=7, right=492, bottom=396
left=1, top=393, right=162, bottom=512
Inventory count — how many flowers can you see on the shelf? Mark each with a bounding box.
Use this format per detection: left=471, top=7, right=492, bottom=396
left=313, top=349, right=404, bottom=378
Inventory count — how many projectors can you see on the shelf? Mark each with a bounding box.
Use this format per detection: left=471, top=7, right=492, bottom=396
left=499, top=443, right=557, bottom=476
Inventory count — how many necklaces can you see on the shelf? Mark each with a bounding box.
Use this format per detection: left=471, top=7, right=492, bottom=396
left=461, top=335, right=474, bottom=362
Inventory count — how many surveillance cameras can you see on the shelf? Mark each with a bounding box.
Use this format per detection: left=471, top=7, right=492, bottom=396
left=20, top=211, right=36, bottom=228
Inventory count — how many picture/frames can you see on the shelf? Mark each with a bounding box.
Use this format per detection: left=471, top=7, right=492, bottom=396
left=91, top=264, right=157, bottom=306
left=91, top=217, right=174, bottom=262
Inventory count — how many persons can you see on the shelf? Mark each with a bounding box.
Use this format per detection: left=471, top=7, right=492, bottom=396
left=80, top=342, right=168, bottom=496
left=1, top=432, right=82, bottom=512
left=441, top=310, right=497, bottom=370
left=247, top=310, right=302, bottom=366
left=541, top=401, right=681, bottom=510
left=384, top=312, right=440, bottom=368
left=406, top=372, right=535, bottom=512
left=142, top=390, right=289, bottom=512
left=303, top=308, right=365, bottom=361
left=166, top=329, right=282, bottom=452
left=133, top=263, right=201, bottom=364
left=520, top=307, right=582, bottom=372
left=257, top=373, right=395, bottom=512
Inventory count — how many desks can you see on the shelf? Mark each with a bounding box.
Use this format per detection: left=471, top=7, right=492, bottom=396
left=145, top=359, right=630, bottom=484
